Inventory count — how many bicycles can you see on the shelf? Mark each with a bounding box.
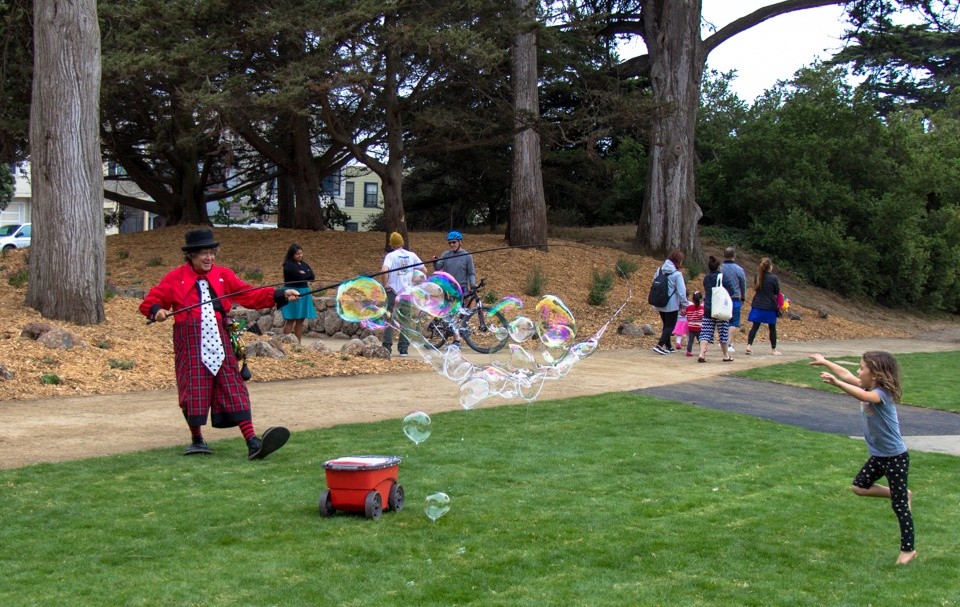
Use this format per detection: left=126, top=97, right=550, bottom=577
left=423, top=277, right=510, bottom=354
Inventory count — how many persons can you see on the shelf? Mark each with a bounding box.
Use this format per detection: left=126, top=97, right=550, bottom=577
left=381, top=231, right=427, bottom=358
left=651, top=249, right=688, bottom=354
left=809, top=350, right=917, bottom=566
left=746, top=257, right=783, bottom=356
left=698, top=255, right=734, bottom=361
left=139, top=225, right=302, bottom=460
left=671, top=289, right=695, bottom=349
left=718, top=247, right=746, bottom=352
left=680, top=290, right=708, bottom=357
left=279, top=243, right=317, bottom=345
left=431, top=231, right=476, bottom=350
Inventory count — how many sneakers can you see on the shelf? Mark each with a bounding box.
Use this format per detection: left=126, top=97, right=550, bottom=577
left=666, top=347, right=676, bottom=353
left=654, top=344, right=667, bottom=355
left=399, top=350, right=408, bottom=357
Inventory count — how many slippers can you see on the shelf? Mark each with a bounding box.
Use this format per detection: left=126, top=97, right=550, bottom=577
left=722, top=354, right=735, bottom=362
left=771, top=350, right=783, bottom=356
left=746, top=348, right=753, bottom=356
left=698, top=356, right=706, bottom=362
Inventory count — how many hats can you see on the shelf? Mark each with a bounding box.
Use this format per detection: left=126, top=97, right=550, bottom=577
left=388, top=232, right=404, bottom=248
left=180, top=227, right=220, bottom=253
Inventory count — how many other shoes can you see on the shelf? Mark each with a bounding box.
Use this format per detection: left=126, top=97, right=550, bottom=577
left=676, top=343, right=682, bottom=349
left=686, top=352, right=695, bottom=356
left=703, top=348, right=708, bottom=353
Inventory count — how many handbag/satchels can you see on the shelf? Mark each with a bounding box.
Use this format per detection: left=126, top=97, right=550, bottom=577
left=709, top=273, right=734, bottom=322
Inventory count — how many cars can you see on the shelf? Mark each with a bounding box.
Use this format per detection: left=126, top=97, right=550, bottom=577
left=0, top=222, right=32, bottom=257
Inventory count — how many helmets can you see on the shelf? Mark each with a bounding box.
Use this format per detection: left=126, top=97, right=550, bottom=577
left=448, top=232, right=463, bottom=241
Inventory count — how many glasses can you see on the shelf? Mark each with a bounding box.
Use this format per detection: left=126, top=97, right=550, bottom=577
left=448, top=241, right=459, bottom=244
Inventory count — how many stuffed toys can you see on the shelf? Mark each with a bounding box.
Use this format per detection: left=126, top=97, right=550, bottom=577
left=776, top=291, right=791, bottom=314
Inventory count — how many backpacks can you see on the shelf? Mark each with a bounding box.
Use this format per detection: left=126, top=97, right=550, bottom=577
left=647, top=265, right=679, bottom=308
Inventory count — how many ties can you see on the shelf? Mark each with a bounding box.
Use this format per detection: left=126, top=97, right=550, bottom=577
left=197, top=279, right=224, bottom=379
